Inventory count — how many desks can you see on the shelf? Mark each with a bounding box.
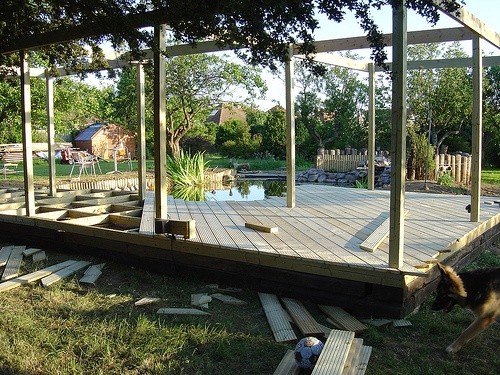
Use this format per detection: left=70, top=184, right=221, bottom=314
left=105, top=147, right=126, bottom=175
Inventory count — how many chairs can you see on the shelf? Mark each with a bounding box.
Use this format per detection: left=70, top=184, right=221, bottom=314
left=69, top=151, right=102, bottom=181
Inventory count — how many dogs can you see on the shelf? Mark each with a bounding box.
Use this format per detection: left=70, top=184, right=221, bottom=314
left=431, top=262, right=500, bottom=354
